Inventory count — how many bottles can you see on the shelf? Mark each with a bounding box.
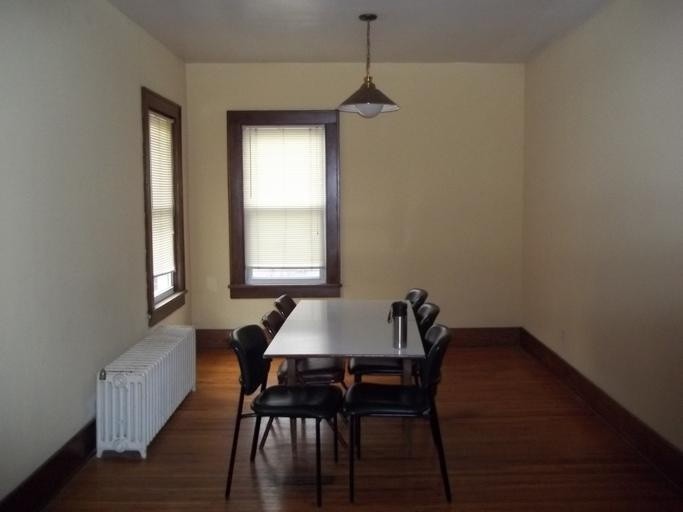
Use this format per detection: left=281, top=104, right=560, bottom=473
left=392, top=301, right=407, bottom=350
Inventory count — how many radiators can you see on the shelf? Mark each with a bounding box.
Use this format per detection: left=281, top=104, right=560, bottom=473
left=95, top=323, right=198, bottom=460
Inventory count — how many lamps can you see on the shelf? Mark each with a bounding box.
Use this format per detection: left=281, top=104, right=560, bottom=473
left=334, top=13, right=400, bottom=119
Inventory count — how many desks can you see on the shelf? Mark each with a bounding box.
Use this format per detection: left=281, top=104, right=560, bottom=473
left=263, top=296, right=425, bottom=448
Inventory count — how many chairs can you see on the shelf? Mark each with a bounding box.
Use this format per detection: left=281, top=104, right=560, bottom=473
left=347, top=323, right=452, bottom=506
left=228, top=323, right=345, bottom=507
left=275, top=293, right=296, bottom=318
left=263, top=310, right=350, bottom=428
left=348, top=301, right=441, bottom=385
left=405, top=286, right=429, bottom=314
